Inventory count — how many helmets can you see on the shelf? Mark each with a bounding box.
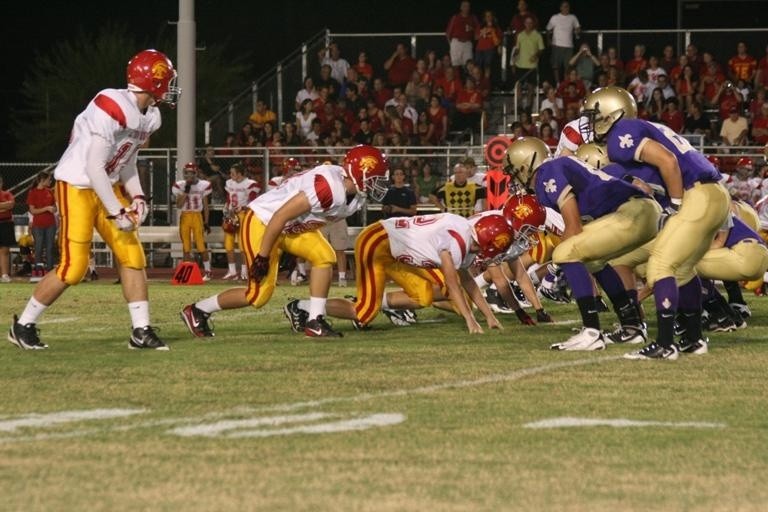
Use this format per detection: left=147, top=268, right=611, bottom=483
left=474, top=137, right=551, bottom=267
left=707, top=155, right=721, bottom=166
left=283, top=158, right=301, bottom=175
left=344, top=144, right=389, bottom=202
left=184, top=163, right=199, bottom=176
left=576, top=86, right=638, bottom=170
left=127, top=49, right=183, bottom=109
left=223, top=219, right=239, bottom=234
left=736, top=158, right=753, bottom=170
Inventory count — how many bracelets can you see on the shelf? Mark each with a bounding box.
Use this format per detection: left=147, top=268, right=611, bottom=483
left=670, top=197, right=683, bottom=209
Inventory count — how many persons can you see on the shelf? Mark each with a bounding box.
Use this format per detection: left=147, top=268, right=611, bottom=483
left=182, top=143, right=392, bottom=339
left=504, top=136, right=660, bottom=352
left=1, top=2, right=768, bottom=288
left=284, top=213, right=515, bottom=334
left=696, top=212, right=768, bottom=330
left=569, top=144, right=670, bottom=332
left=580, top=86, right=731, bottom=361
left=380, top=196, right=556, bottom=328
left=9, top=50, right=181, bottom=349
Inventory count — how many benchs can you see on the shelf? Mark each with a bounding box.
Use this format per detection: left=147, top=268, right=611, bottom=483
left=679, top=97, right=721, bottom=148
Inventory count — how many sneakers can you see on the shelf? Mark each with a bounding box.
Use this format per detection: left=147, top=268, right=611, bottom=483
left=7, top=314, right=48, bottom=350
left=222, top=273, right=239, bottom=281
left=624, top=340, right=681, bottom=362
left=1, top=274, right=12, bottom=283
left=703, top=302, right=751, bottom=333
left=383, top=308, right=416, bottom=326
left=127, top=326, right=169, bottom=351
left=304, top=315, right=344, bottom=338
left=345, top=295, right=370, bottom=330
left=485, top=281, right=532, bottom=314
left=677, top=336, right=709, bottom=355
left=179, top=303, right=214, bottom=338
left=202, top=270, right=213, bottom=281
left=283, top=299, right=309, bottom=332
left=550, top=322, right=649, bottom=352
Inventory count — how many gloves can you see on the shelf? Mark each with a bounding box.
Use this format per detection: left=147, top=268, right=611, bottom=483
left=185, top=183, right=191, bottom=194
left=114, top=196, right=148, bottom=232
left=536, top=309, right=554, bottom=321
left=250, top=254, right=270, bottom=283
left=203, top=223, right=211, bottom=235
left=516, top=308, right=536, bottom=327
left=659, top=198, right=682, bottom=229
left=540, top=264, right=571, bottom=304
